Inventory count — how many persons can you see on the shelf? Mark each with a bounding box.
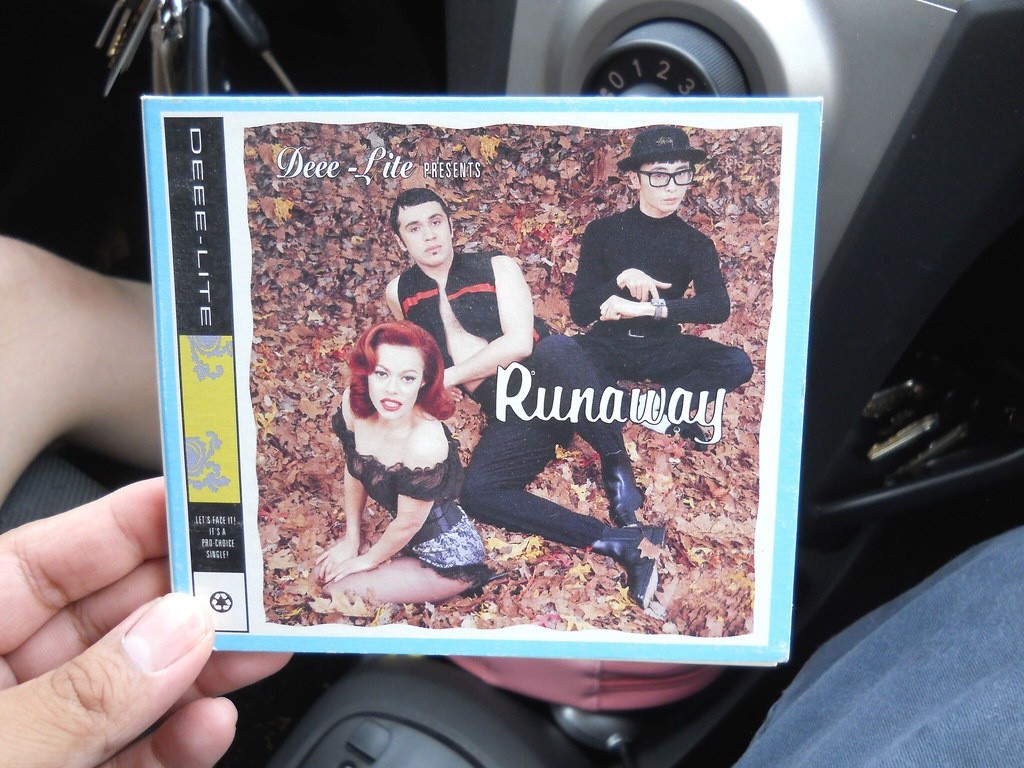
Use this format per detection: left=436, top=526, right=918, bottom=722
left=314, top=320, right=494, bottom=613
left=570, top=127, right=754, bottom=450
left=0, top=233, right=164, bottom=530
left=0, top=473, right=1023, bottom=767
left=385, top=187, right=666, bottom=611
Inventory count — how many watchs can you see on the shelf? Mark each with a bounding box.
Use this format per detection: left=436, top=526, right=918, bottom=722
left=651, top=297, right=665, bottom=321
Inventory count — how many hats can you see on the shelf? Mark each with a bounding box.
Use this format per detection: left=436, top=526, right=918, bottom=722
left=616, top=126, right=707, bottom=171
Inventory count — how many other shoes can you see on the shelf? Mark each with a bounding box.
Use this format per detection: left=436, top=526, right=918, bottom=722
left=665, top=418, right=708, bottom=452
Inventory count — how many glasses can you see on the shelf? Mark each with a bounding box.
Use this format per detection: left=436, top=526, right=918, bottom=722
left=637, top=168, right=696, bottom=189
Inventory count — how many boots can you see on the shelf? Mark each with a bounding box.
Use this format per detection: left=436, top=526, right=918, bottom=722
left=600, top=434, right=645, bottom=528
left=590, top=523, right=667, bottom=610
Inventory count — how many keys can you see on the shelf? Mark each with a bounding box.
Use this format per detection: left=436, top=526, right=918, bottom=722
left=92, top=0, right=197, bottom=101
